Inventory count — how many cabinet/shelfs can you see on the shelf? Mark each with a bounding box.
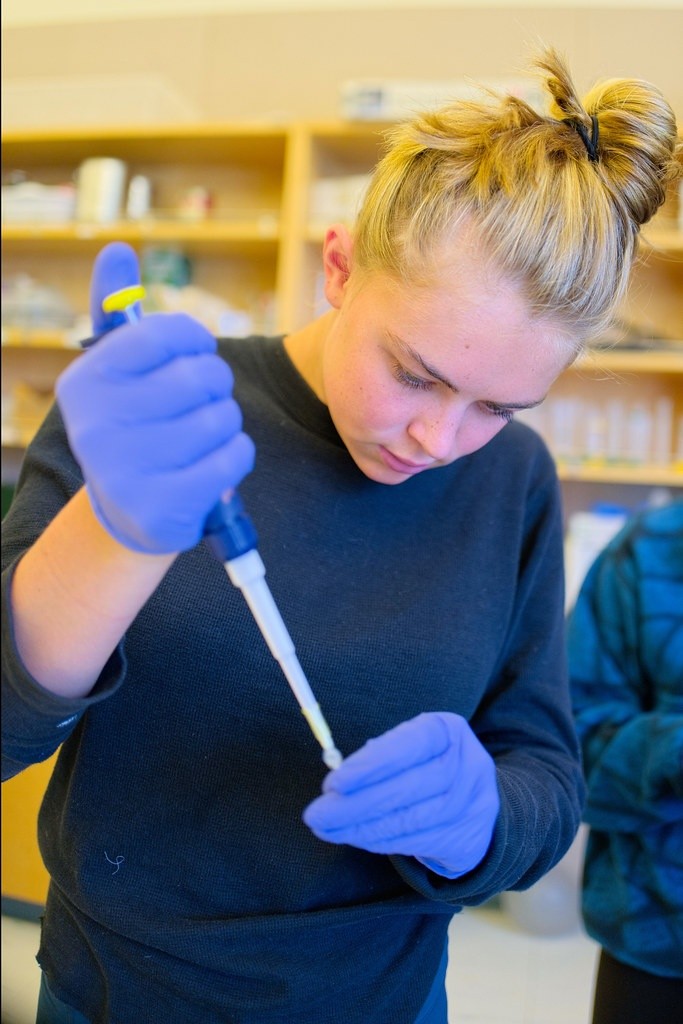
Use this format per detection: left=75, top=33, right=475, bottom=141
left=0, top=124, right=683, bottom=494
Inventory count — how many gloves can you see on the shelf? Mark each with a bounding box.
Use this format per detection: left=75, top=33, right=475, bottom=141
left=55, top=243, right=256, bottom=554
left=302, top=712, right=500, bottom=879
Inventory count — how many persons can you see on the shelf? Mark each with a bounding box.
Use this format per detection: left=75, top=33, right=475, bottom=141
left=0, top=48, right=676, bottom=1024
left=566, top=504, right=683, bottom=1024
